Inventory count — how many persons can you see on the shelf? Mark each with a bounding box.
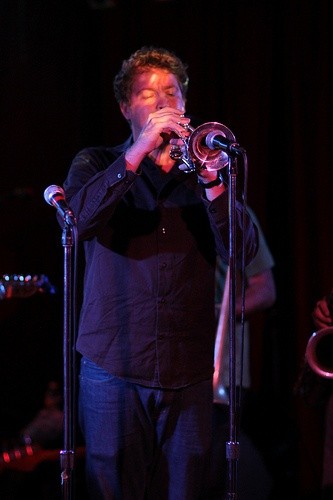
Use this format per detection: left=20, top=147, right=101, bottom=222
left=312, top=298, right=331, bottom=331
left=58, top=52, right=258, bottom=500
left=213, top=198, right=275, bottom=500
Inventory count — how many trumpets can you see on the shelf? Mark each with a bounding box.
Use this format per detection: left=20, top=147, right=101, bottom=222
left=169, top=114, right=235, bottom=174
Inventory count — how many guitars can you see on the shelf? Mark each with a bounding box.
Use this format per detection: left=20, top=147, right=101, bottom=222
left=1, top=443, right=85, bottom=473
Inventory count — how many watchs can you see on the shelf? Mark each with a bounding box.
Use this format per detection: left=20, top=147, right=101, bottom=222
left=198, top=172, right=224, bottom=189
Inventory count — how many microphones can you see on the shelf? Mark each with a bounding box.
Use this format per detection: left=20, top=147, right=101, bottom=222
left=206, top=130, right=242, bottom=157
left=44, top=185, right=77, bottom=228
